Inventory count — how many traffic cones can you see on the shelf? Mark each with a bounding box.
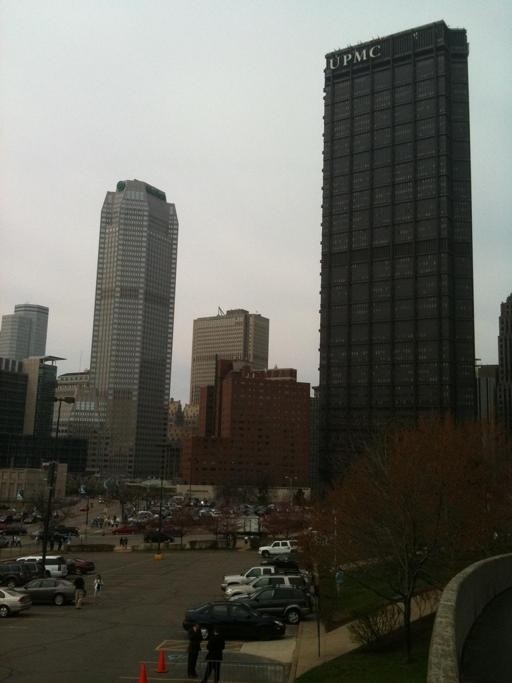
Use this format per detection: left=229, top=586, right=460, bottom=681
left=155, top=648, right=169, bottom=673
left=138, top=663, right=147, bottom=683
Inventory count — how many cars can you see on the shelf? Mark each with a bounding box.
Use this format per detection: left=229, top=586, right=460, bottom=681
left=0, top=555, right=95, bottom=618
left=1, top=496, right=223, bottom=547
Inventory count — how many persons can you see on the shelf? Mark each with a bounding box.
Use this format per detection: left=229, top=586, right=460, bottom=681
left=49, top=536, right=55, bottom=551
left=120, top=536, right=124, bottom=547
left=201, top=626, right=226, bottom=683
left=72, top=572, right=88, bottom=608
left=123, top=536, right=128, bottom=547
left=187, top=623, right=203, bottom=678
left=92, top=574, right=105, bottom=603
left=16, top=537, right=22, bottom=546
left=58, top=536, right=65, bottom=551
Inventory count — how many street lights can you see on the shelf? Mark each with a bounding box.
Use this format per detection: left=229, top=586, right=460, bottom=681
left=284, top=476, right=298, bottom=505
left=43, top=397, right=75, bottom=573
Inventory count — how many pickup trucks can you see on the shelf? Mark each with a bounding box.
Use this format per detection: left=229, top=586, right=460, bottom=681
left=182, top=540, right=313, bottom=640
